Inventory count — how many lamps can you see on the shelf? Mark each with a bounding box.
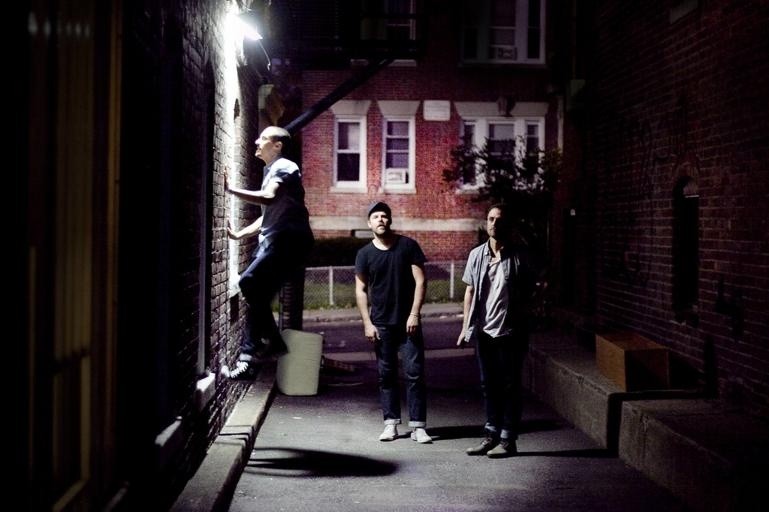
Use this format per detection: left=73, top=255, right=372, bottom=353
left=234, top=0, right=271, bottom=42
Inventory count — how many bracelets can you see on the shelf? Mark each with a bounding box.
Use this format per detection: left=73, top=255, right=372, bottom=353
left=408, top=312, right=421, bottom=317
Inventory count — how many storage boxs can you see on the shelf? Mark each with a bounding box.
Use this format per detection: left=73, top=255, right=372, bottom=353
left=595, top=330, right=672, bottom=394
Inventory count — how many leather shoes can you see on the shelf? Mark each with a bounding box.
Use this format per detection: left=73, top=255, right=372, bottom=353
left=466, top=437, right=517, bottom=458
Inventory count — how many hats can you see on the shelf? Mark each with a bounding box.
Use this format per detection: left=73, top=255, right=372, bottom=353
left=367, top=202, right=391, bottom=217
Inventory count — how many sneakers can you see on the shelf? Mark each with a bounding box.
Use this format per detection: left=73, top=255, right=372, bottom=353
left=379, top=425, right=398, bottom=441
left=261, top=339, right=288, bottom=358
left=221, top=362, right=256, bottom=381
left=411, top=429, right=432, bottom=443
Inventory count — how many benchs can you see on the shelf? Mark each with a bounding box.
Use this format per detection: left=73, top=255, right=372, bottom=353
left=521, top=331, right=707, bottom=454
left=618, top=396, right=768, bottom=511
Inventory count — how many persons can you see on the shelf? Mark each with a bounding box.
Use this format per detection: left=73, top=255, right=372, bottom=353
left=354, top=201, right=433, bottom=443
left=223, top=125, right=314, bottom=379
left=455, top=203, right=532, bottom=458
